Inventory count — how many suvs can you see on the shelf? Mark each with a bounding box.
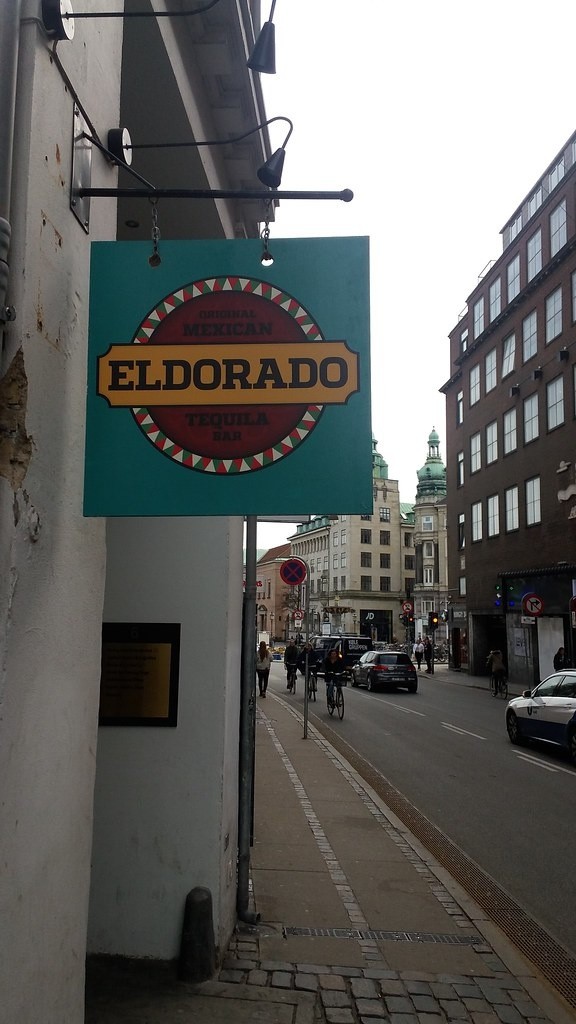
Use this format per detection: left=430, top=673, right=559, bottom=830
left=504, top=668, right=576, bottom=764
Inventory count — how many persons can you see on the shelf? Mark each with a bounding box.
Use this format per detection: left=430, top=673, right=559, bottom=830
left=322, top=648, right=348, bottom=704
left=284, top=640, right=299, bottom=688
left=553, top=647, right=564, bottom=671
left=299, top=642, right=319, bottom=692
left=257, top=641, right=273, bottom=698
left=415, top=636, right=432, bottom=673
left=486, top=647, right=507, bottom=696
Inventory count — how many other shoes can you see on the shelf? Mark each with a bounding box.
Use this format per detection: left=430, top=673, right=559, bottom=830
left=262, top=691, right=266, bottom=698
left=259, top=692, right=262, bottom=696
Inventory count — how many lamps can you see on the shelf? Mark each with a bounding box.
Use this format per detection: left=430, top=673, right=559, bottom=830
left=509, top=384, right=520, bottom=397
left=557, top=347, right=568, bottom=361
left=531, top=366, right=543, bottom=380
left=41, top=0, right=276, bottom=75
left=107, top=117, right=293, bottom=187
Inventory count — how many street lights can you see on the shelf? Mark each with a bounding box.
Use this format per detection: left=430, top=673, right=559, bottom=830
left=269, top=612, right=275, bottom=637
left=351, top=613, right=357, bottom=635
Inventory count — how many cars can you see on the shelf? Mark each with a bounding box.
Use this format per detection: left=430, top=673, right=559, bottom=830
left=350, top=650, right=418, bottom=694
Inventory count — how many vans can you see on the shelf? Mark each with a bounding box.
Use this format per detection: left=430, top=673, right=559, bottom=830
left=298, top=632, right=373, bottom=676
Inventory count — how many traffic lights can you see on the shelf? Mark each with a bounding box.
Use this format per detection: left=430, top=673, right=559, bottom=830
left=408, top=613, right=414, bottom=627
left=431, top=613, right=438, bottom=629
left=402, top=612, right=408, bottom=627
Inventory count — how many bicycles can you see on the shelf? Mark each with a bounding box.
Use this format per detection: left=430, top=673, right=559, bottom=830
left=284, top=662, right=298, bottom=695
left=372, top=640, right=449, bottom=662
left=482, top=668, right=509, bottom=700
left=325, top=671, right=350, bottom=720
left=301, top=659, right=323, bottom=703
